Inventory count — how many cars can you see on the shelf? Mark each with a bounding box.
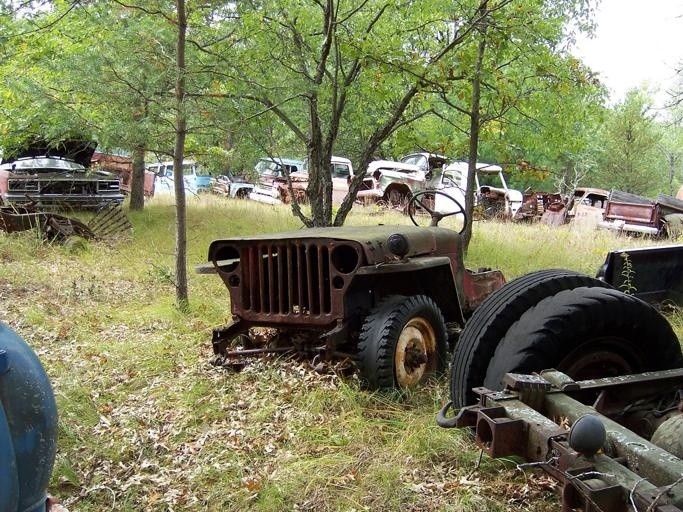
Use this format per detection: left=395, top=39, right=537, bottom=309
left=0, top=157, right=125, bottom=232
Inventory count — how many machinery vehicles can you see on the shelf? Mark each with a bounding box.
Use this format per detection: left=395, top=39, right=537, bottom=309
left=194, top=192, right=510, bottom=399
